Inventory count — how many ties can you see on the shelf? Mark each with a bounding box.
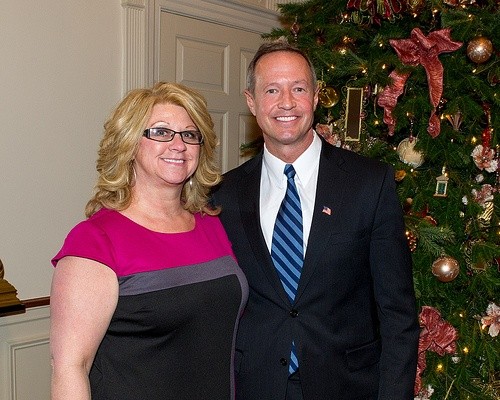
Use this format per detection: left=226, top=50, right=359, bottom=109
left=271, top=163, right=304, bottom=374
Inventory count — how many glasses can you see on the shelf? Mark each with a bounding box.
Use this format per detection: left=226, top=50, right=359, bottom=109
left=140, top=127, right=206, bottom=146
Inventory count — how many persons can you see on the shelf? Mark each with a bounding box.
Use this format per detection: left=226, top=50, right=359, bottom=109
left=43, top=79, right=252, bottom=400
left=206, top=37, right=421, bottom=400
